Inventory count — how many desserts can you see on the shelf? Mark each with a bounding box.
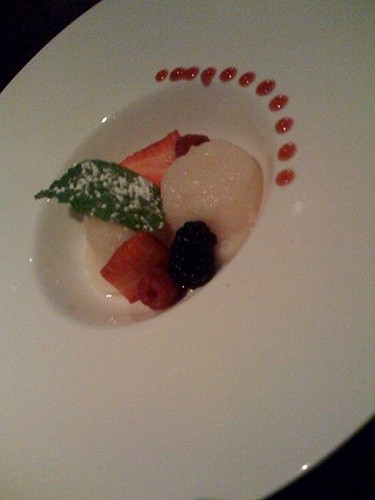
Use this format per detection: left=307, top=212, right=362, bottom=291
left=32, top=128, right=264, bottom=311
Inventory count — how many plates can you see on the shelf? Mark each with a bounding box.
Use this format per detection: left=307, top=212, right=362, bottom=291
left=1, top=0, right=375, bottom=499
